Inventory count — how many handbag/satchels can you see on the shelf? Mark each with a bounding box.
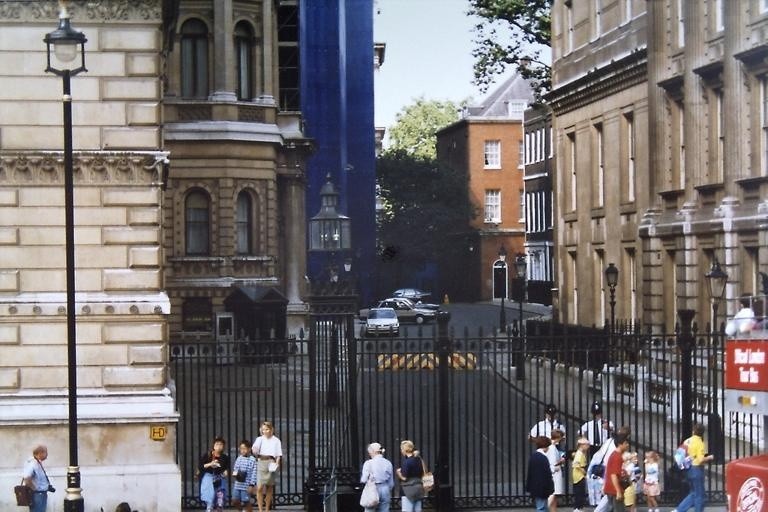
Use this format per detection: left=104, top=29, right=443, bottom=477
left=402, top=478, right=427, bottom=503
left=358, top=478, right=381, bottom=508
left=13, top=483, right=34, bottom=508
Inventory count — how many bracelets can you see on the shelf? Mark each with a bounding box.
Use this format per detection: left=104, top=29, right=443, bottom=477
left=249, top=484, right=254, bottom=486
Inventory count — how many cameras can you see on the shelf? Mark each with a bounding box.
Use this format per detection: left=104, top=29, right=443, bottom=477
left=48, top=484, right=55, bottom=492
left=236, top=471, right=247, bottom=482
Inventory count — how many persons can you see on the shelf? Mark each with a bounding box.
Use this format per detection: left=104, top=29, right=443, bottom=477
left=230, top=439, right=258, bottom=511
left=577, top=400, right=616, bottom=461
left=733, top=298, right=757, bottom=322
left=670, top=420, right=716, bottom=511
left=22, top=443, right=51, bottom=511
left=251, top=420, right=283, bottom=512
left=527, top=404, right=568, bottom=449
left=527, top=426, right=663, bottom=512
left=359, top=441, right=396, bottom=512
left=395, top=438, right=428, bottom=512
left=198, top=437, right=232, bottom=511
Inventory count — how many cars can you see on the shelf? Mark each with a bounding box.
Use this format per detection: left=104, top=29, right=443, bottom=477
left=365, top=285, right=449, bottom=335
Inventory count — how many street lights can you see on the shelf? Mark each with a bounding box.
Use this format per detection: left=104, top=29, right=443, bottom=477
left=45, top=2, right=92, bottom=509
left=513, top=253, right=528, bottom=380
left=604, top=262, right=621, bottom=368
left=306, top=174, right=355, bottom=512
left=704, top=255, right=729, bottom=463
left=497, top=242, right=508, bottom=333
